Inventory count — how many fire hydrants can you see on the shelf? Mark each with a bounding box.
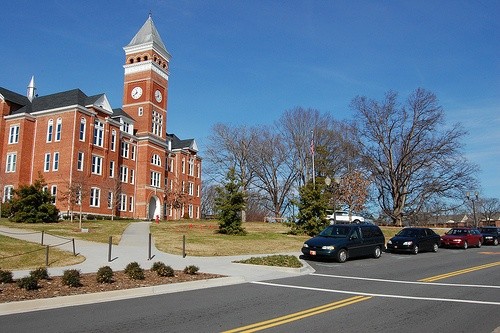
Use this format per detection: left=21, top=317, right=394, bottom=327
left=155, top=215, right=159, bottom=224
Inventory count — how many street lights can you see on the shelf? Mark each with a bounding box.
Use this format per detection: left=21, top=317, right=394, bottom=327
left=325, top=173, right=341, bottom=224
left=466, top=191, right=479, bottom=229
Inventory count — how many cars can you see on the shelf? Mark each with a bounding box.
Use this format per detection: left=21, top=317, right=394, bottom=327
left=387, top=227, right=442, bottom=255
left=440, top=227, right=483, bottom=249
left=325, top=209, right=365, bottom=226
left=301, top=223, right=385, bottom=263
left=481, top=226, right=500, bottom=246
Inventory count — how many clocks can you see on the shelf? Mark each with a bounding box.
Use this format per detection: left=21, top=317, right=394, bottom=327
left=154, top=89, right=163, bottom=104
left=130, top=86, right=143, bottom=100
